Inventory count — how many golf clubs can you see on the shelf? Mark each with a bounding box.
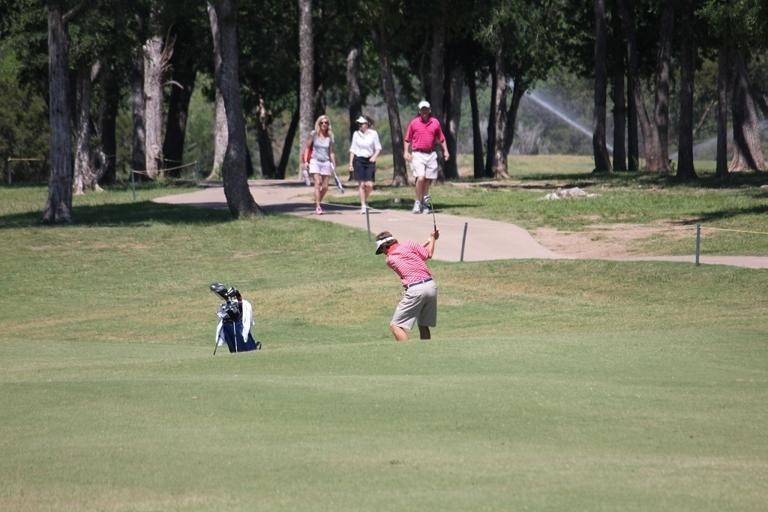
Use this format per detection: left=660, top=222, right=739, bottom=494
left=422, top=195, right=437, bottom=232
left=208, top=284, right=242, bottom=355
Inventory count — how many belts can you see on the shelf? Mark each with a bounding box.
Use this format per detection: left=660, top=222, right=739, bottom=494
left=417, top=150, right=434, bottom=153
left=409, top=278, right=432, bottom=287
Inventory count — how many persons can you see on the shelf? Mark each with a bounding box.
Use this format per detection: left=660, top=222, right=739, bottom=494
left=374, top=229, right=440, bottom=341
left=302, top=114, right=337, bottom=215
left=214, top=288, right=260, bottom=351
left=348, top=115, right=382, bottom=213
left=403, top=100, right=450, bottom=216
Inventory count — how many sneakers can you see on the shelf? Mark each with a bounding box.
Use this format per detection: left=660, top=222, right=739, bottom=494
left=361, top=203, right=369, bottom=213
left=316, top=207, right=322, bottom=215
left=413, top=200, right=429, bottom=214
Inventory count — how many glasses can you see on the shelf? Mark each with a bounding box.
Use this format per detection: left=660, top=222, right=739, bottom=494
left=320, top=122, right=328, bottom=124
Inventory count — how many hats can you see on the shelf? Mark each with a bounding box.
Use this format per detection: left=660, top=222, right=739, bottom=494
left=356, top=116, right=371, bottom=125
left=418, top=100, right=430, bottom=109
left=376, top=237, right=394, bottom=254
